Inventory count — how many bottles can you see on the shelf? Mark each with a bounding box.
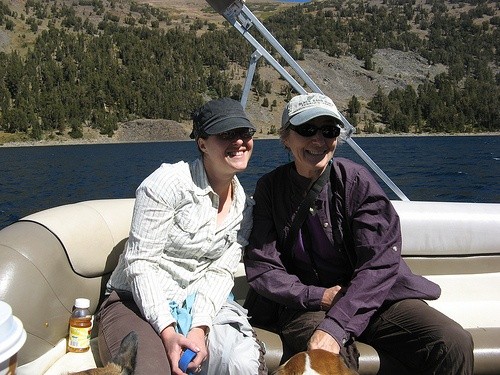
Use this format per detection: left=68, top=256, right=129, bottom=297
left=67, top=298, right=92, bottom=354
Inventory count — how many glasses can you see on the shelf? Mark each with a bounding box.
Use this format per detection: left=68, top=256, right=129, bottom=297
left=218, top=128, right=254, bottom=140
left=290, top=123, right=341, bottom=139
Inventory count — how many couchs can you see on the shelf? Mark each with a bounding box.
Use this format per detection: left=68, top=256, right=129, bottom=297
left=1, top=198, right=500, bottom=375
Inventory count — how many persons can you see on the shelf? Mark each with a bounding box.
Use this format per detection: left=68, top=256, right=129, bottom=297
left=97, top=96, right=269, bottom=375
left=243, top=90, right=475, bottom=375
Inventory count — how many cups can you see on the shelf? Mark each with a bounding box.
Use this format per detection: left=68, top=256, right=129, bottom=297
left=0, top=299, right=27, bottom=374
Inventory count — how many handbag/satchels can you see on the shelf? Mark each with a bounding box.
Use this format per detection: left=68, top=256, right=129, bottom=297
left=245, top=292, right=284, bottom=330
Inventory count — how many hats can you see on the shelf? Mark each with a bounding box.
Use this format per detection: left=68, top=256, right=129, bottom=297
left=280, top=92, right=345, bottom=127
left=191, top=97, right=255, bottom=135
